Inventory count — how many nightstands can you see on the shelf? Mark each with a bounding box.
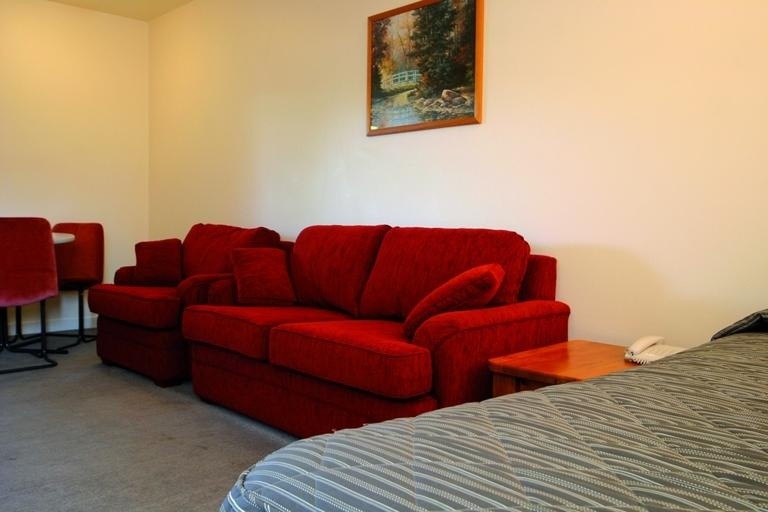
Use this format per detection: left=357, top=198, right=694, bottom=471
left=487, top=339, right=638, bottom=398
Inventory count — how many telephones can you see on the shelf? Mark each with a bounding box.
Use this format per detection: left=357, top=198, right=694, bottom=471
left=624, top=335, right=688, bottom=364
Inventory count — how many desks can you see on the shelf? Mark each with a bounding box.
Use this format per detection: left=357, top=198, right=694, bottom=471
left=51, top=230, right=76, bottom=354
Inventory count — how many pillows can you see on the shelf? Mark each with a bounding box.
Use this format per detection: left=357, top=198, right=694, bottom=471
left=359, top=225, right=530, bottom=321
left=227, top=247, right=296, bottom=305
left=181, top=224, right=281, bottom=279
left=133, top=238, right=182, bottom=283
left=286, top=226, right=392, bottom=315
left=400, top=262, right=506, bottom=340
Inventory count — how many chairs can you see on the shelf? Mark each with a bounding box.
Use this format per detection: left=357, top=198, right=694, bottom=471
left=11, top=222, right=105, bottom=349
left=88, top=223, right=281, bottom=389
left=0, top=216, right=59, bottom=372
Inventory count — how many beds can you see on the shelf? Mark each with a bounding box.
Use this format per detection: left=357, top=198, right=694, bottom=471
left=218, top=307, right=768, bottom=512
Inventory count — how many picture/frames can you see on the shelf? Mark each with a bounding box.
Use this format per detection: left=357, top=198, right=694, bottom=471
left=365, top=0, right=484, bottom=137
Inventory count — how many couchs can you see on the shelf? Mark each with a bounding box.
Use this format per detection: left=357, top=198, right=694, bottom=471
left=182, top=223, right=570, bottom=439
